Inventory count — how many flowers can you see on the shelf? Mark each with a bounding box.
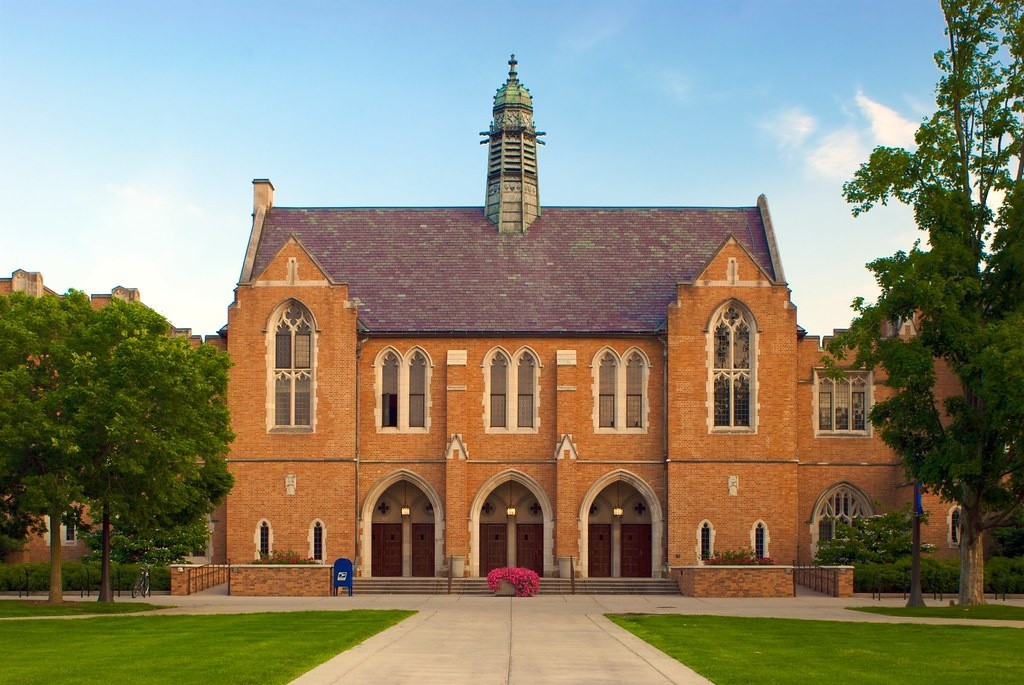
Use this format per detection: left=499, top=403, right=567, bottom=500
left=487, top=567, right=541, bottom=597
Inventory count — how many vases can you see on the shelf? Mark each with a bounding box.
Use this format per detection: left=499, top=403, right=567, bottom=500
left=495, top=580, right=516, bottom=596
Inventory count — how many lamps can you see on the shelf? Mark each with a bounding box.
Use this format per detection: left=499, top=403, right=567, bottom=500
left=506, top=482, right=516, bottom=518
left=401, top=480, right=410, bottom=516
left=613, top=481, right=623, bottom=516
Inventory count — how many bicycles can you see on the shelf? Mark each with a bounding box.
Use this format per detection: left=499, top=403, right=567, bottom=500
left=131, top=563, right=150, bottom=599
